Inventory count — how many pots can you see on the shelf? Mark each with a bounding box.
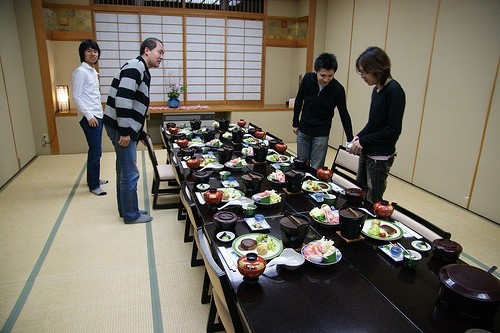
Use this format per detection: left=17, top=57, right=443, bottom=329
left=435, top=263, right=500, bottom=328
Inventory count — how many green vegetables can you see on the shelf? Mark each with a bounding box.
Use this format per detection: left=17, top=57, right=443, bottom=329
left=256, top=234, right=276, bottom=251
left=315, top=214, right=326, bottom=222
left=322, top=251, right=337, bottom=263
left=367, top=224, right=383, bottom=236
left=197, top=124, right=277, bottom=203
left=310, top=184, right=323, bottom=191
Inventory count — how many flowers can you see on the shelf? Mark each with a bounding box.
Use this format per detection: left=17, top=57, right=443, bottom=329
left=166, top=84, right=186, bottom=98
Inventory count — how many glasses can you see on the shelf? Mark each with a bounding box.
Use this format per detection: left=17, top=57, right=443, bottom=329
left=356, top=70, right=367, bottom=75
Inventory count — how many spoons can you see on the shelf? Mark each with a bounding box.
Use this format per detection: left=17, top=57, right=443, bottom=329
left=218, top=200, right=242, bottom=211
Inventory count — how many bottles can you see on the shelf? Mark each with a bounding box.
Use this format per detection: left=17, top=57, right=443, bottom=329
left=204, top=187, right=221, bottom=206
left=274, top=141, right=287, bottom=153
left=254, top=128, right=266, bottom=139
left=176, top=135, right=188, bottom=148
left=372, top=199, right=394, bottom=218
left=317, top=165, right=334, bottom=181
left=238, top=119, right=246, bottom=126
left=185, top=156, right=199, bottom=169
left=169, top=127, right=178, bottom=133
left=238, top=252, right=266, bottom=283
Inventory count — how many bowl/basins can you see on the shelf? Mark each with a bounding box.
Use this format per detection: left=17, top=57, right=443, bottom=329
left=432, top=238, right=462, bottom=265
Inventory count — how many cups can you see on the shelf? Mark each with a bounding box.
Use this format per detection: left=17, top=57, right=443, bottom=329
left=402, top=249, right=422, bottom=270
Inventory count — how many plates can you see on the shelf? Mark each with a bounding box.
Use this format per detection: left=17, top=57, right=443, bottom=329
left=165, top=122, right=431, bottom=270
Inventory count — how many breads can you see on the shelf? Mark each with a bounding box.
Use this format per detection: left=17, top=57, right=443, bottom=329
left=380, top=225, right=397, bottom=235
left=241, top=239, right=257, bottom=250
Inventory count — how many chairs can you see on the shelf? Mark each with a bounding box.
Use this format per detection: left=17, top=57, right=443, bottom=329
left=138, top=126, right=245, bottom=333
left=248, top=123, right=452, bottom=242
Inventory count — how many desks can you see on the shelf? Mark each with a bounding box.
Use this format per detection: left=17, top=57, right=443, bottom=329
left=165, top=127, right=373, bottom=224
left=203, top=214, right=422, bottom=333
left=55, top=104, right=302, bottom=150
left=300, top=208, right=500, bottom=333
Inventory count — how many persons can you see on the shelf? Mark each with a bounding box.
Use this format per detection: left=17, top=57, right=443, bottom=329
left=103, top=37, right=165, bottom=223
left=351, top=47, right=406, bottom=202
left=292, top=52, right=354, bottom=170
left=72, top=39, right=109, bottom=195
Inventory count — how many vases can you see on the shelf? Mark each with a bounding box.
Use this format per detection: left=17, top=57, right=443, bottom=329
left=167, top=96, right=180, bottom=108
